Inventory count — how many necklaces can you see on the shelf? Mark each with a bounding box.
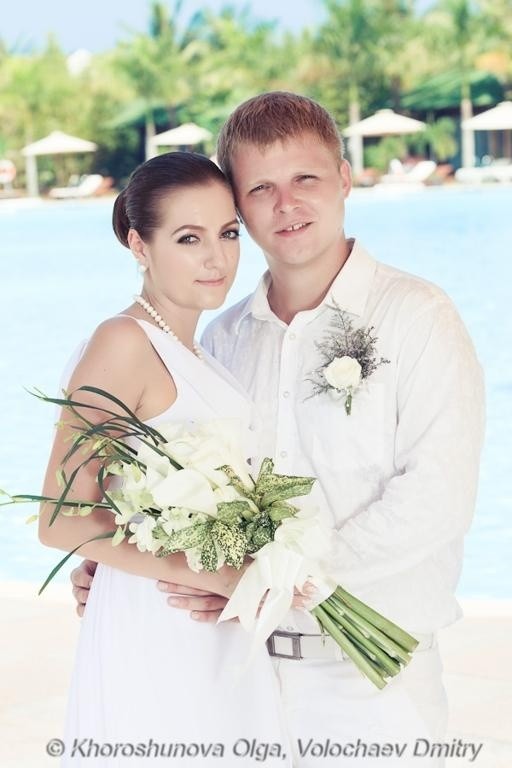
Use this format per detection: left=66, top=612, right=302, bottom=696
left=135, top=292, right=207, bottom=377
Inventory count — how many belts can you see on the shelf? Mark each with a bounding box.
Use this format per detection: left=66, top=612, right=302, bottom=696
left=265, top=629, right=355, bottom=662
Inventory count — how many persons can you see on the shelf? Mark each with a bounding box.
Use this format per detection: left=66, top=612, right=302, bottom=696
left=70, top=92, right=487, bottom=697
left=39, top=154, right=314, bottom=665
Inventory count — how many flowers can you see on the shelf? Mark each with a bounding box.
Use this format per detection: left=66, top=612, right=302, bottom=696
left=2, top=377, right=419, bottom=692
left=311, top=303, right=383, bottom=414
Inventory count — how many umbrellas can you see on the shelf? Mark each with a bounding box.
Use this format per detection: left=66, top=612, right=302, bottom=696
left=21, top=128, right=100, bottom=198
left=340, top=107, right=426, bottom=177
left=148, top=122, right=213, bottom=155
left=462, top=100, right=512, bottom=164
